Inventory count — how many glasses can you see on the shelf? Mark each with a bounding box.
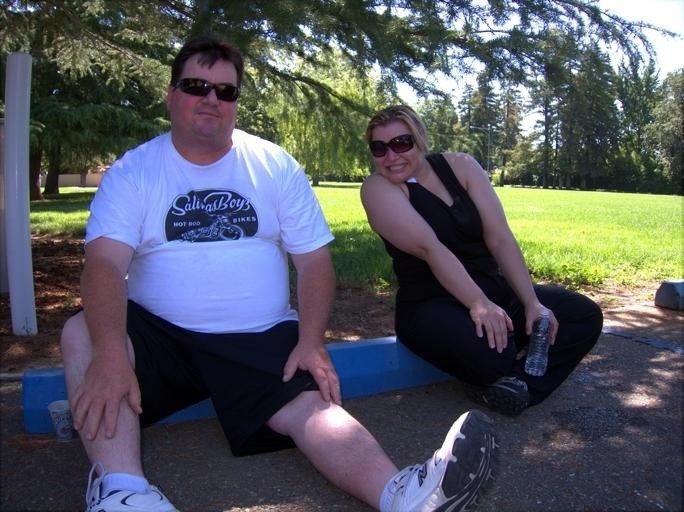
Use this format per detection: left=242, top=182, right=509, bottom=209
left=369, top=134, right=414, bottom=157
left=174, top=78, right=240, bottom=102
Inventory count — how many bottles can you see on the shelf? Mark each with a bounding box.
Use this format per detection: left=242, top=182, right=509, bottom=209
left=524, top=309, right=553, bottom=377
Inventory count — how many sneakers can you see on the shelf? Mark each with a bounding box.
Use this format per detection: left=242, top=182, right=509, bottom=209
left=392, top=407, right=497, bottom=512
left=87, top=480, right=179, bottom=512
left=467, top=376, right=529, bottom=416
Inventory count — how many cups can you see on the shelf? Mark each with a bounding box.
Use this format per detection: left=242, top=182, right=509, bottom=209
left=48, top=399, right=74, bottom=443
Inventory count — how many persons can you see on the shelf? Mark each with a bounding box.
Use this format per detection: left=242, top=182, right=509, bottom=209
left=57, top=34, right=503, bottom=512
left=360, top=103, right=605, bottom=420
